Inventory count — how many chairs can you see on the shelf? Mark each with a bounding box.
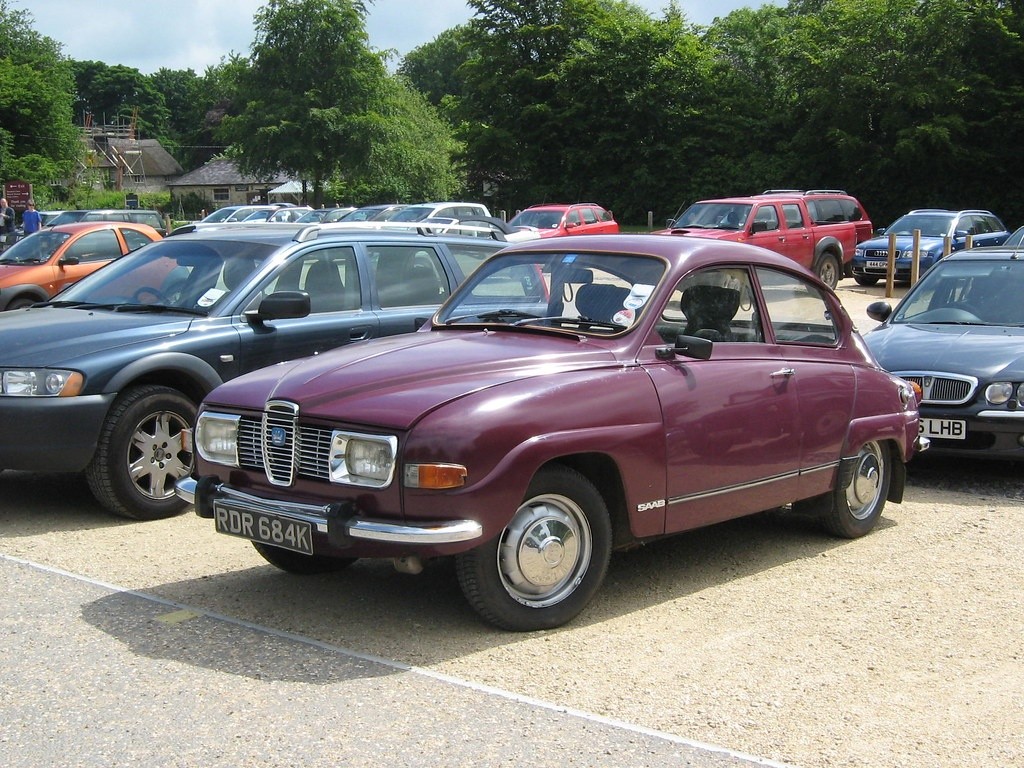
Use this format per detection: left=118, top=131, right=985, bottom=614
left=681, top=283, right=740, bottom=348
left=574, top=282, right=641, bottom=332
left=309, top=259, right=346, bottom=308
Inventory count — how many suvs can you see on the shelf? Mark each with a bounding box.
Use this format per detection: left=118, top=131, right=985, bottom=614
left=501, top=202, right=619, bottom=256
left=0, top=221, right=562, bottom=522
left=852, top=208, right=1011, bottom=287
left=34, top=210, right=170, bottom=252
left=718, top=187, right=875, bottom=278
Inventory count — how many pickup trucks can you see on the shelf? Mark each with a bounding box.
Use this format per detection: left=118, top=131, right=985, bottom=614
left=649, top=193, right=858, bottom=296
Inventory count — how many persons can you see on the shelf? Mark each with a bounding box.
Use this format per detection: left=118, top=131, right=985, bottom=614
left=0, top=198, right=15, bottom=252
left=262, top=196, right=267, bottom=205
left=22, top=200, right=42, bottom=237
left=270, top=196, right=276, bottom=204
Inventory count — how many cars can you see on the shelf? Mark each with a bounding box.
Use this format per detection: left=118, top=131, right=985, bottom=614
left=999, top=226, right=1024, bottom=253
left=196, top=202, right=496, bottom=240
left=860, top=245, right=1024, bottom=465
left=0, top=221, right=196, bottom=319
left=170, top=232, right=931, bottom=634
